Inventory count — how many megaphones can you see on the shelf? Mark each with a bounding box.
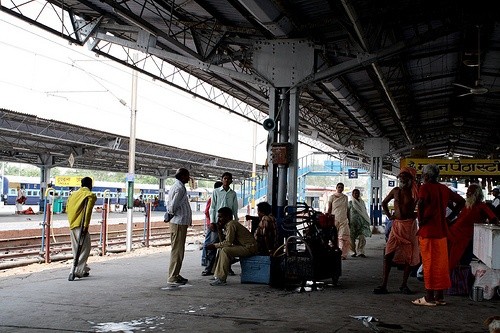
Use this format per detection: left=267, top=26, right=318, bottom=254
left=262, top=118, right=277, bottom=132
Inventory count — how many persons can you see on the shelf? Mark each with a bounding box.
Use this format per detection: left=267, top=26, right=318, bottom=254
left=122, top=198, right=159, bottom=211
left=16, top=187, right=23, bottom=213
left=163, top=168, right=192, bottom=285
left=328, top=163, right=500, bottom=307
left=65, top=177, right=97, bottom=279
left=202, top=172, right=279, bottom=287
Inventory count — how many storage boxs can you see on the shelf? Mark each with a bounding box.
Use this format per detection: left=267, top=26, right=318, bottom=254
left=242, top=251, right=284, bottom=284
left=472, top=223, right=500, bottom=267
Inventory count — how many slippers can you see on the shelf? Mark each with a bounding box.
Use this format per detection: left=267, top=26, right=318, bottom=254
left=411, top=295, right=448, bottom=307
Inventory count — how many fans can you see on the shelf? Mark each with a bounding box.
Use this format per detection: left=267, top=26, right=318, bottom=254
left=451, top=27, right=499, bottom=96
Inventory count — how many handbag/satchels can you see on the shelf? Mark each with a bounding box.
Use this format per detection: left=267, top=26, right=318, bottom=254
left=446, top=265, right=473, bottom=295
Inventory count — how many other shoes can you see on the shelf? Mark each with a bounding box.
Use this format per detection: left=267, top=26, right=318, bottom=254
left=208, top=277, right=217, bottom=280
left=341, top=256, right=346, bottom=260
left=202, top=271, right=214, bottom=276
left=400, top=286, right=413, bottom=295
left=358, top=254, right=366, bottom=258
left=209, top=279, right=228, bottom=286
left=75, top=272, right=91, bottom=278
left=351, top=253, right=357, bottom=257
left=229, top=271, right=235, bottom=276
left=374, top=286, right=388, bottom=294
left=167, top=275, right=188, bottom=287
